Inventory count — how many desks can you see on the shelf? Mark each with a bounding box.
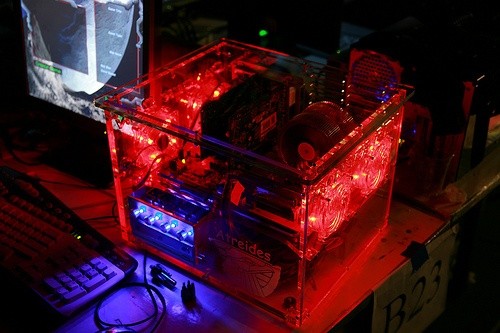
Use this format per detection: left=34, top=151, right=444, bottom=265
left=0, top=43, right=500, bottom=333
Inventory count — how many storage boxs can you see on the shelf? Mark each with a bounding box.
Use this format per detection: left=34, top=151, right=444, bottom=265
left=92, top=40, right=415, bottom=331
left=348, top=225, right=460, bottom=333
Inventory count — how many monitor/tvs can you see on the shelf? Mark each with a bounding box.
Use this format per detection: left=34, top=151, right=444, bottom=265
left=18, top=0, right=163, bottom=191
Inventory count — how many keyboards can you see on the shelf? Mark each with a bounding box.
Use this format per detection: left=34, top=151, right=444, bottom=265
left=0, top=165, right=138, bottom=316
